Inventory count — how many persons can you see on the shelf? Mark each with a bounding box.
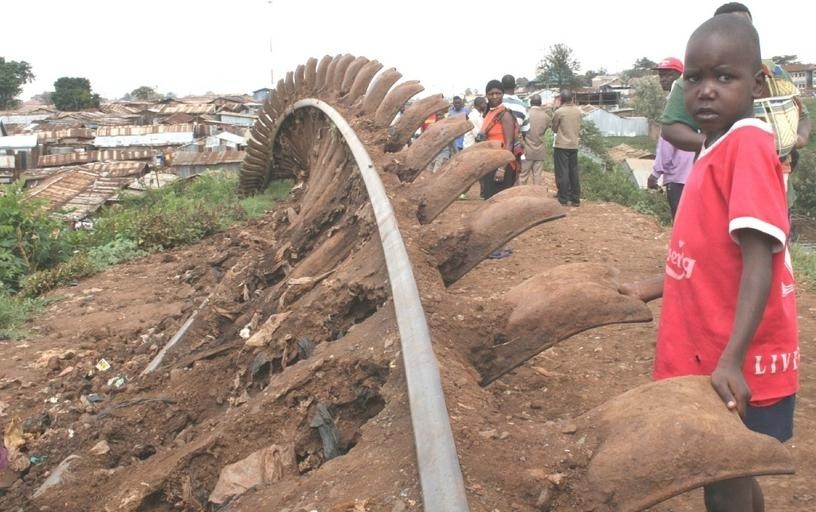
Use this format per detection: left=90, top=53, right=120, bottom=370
left=653, top=13, right=800, bottom=511
left=399, top=75, right=584, bottom=206
left=645, top=57, right=694, bottom=221
left=660, top=0, right=806, bottom=210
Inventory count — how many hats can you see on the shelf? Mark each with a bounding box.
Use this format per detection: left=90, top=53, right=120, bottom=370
left=651, top=57, right=683, bottom=73
